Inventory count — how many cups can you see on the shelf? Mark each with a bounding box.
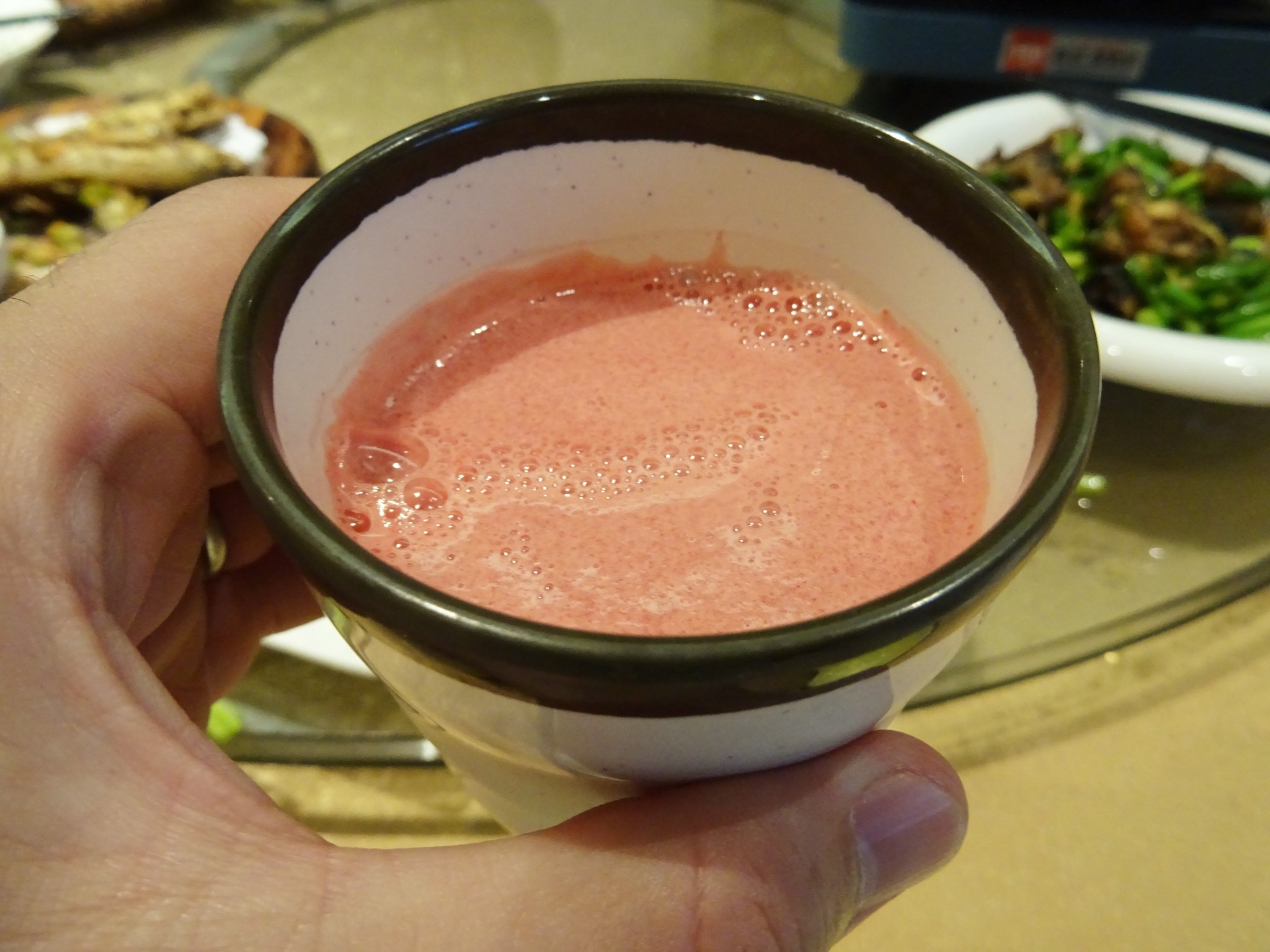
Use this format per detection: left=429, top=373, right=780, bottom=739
left=1137, top=27, right=1268, bottom=109
left=833, top=0, right=1005, bottom=88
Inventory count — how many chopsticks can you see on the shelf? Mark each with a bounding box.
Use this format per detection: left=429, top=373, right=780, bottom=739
left=991, top=75, right=1270, bottom=162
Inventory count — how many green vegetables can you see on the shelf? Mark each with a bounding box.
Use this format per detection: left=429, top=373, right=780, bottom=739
left=980, top=127, right=1270, bottom=337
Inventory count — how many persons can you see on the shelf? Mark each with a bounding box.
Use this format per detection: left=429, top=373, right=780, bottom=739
left=0, top=175, right=969, bottom=952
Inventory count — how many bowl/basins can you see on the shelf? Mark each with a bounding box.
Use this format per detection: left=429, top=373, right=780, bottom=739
left=214, top=74, right=1102, bottom=837
left=0, top=0, right=63, bottom=97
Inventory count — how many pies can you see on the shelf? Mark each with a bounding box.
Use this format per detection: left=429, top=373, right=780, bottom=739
left=0, top=83, right=248, bottom=293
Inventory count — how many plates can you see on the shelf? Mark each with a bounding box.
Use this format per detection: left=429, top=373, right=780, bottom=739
left=904, top=88, right=1270, bottom=409
left=0, top=91, right=319, bottom=192
